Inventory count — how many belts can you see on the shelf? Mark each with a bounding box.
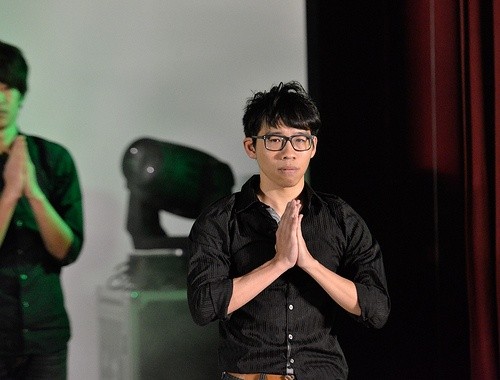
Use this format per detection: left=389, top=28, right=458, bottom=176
left=230, top=372, right=294, bottom=380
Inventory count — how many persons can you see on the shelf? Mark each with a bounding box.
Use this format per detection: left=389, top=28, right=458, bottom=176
left=183, top=79, right=393, bottom=380
left=0, top=40, right=87, bottom=380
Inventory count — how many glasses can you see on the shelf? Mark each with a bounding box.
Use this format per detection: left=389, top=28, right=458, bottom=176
left=252, top=134, right=314, bottom=152
